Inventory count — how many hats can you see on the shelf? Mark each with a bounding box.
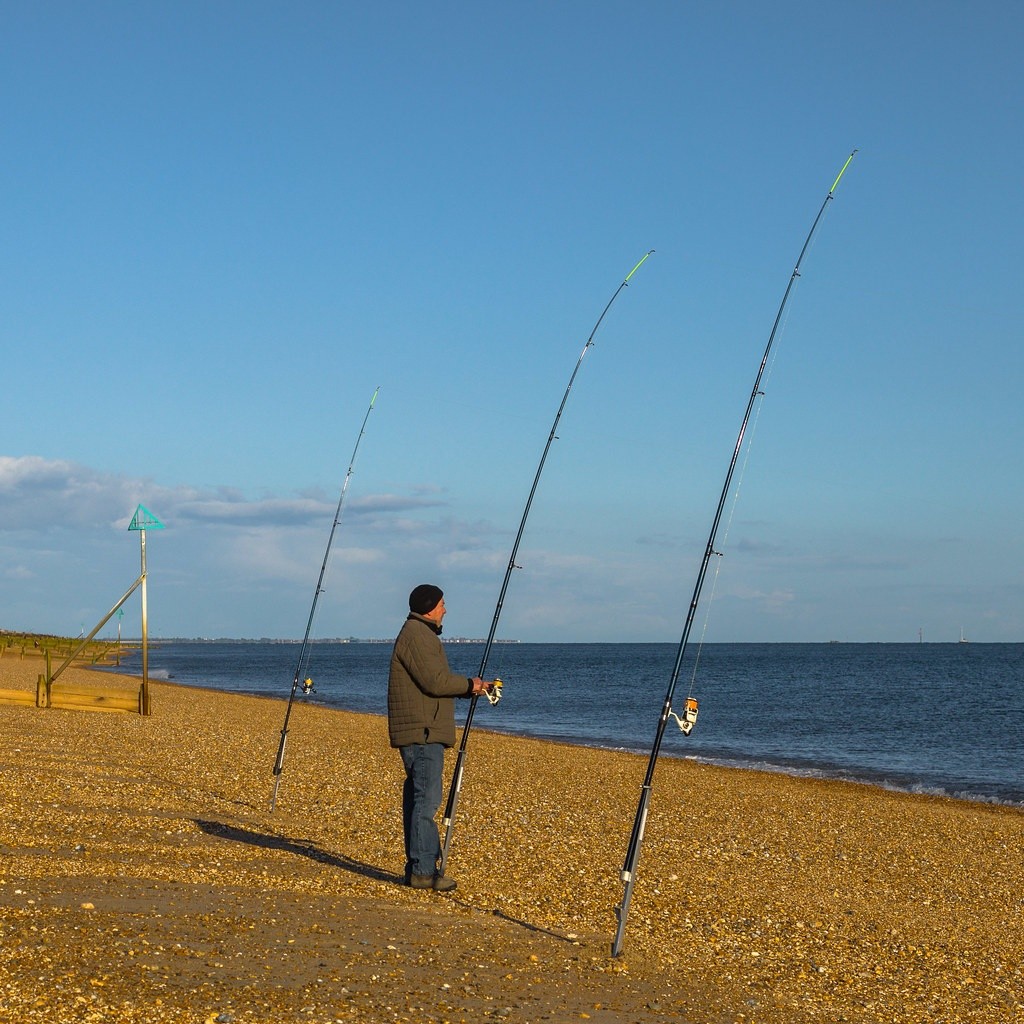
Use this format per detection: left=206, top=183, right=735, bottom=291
left=409, top=584, right=443, bottom=615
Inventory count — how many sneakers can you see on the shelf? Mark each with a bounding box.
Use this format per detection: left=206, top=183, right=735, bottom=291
left=405, top=872, right=457, bottom=891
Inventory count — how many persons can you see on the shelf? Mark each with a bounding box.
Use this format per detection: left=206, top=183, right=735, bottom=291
left=387, top=584, right=487, bottom=891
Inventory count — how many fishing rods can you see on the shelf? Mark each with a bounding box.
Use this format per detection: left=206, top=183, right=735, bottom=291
left=611, top=144, right=864, bottom=955
left=431, top=244, right=658, bottom=888
left=272, top=384, right=383, bottom=774
